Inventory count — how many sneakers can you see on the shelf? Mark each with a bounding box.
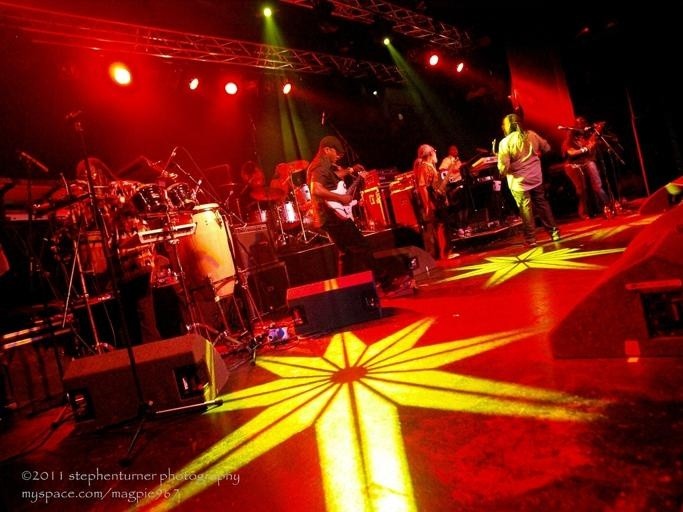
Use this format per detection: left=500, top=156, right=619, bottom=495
left=383, top=281, right=415, bottom=298
left=448, top=253, right=460, bottom=259
left=552, top=227, right=560, bottom=241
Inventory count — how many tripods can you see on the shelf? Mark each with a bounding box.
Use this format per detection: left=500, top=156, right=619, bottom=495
left=284, top=175, right=332, bottom=246
left=1, top=122, right=280, bottom=467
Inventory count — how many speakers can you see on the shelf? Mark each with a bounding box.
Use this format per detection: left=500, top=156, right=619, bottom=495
left=285, top=269, right=381, bottom=337
left=551, top=198, right=683, bottom=357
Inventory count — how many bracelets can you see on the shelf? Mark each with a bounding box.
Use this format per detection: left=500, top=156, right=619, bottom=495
left=581, top=147, right=588, bottom=153
left=347, top=166, right=355, bottom=174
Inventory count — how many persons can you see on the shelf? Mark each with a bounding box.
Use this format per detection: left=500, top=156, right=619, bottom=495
left=77, top=158, right=116, bottom=184
left=244, top=162, right=284, bottom=211
left=411, top=139, right=465, bottom=263
left=496, top=113, right=559, bottom=247
left=560, top=117, right=613, bottom=219
left=306, top=135, right=411, bottom=292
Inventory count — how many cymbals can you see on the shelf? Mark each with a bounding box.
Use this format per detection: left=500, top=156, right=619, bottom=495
left=276, top=159, right=309, bottom=174
left=241, top=160, right=264, bottom=187
left=250, top=187, right=285, bottom=200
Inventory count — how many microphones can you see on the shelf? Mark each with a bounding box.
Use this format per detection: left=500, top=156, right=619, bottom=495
left=558, top=126, right=573, bottom=133
left=584, top=127, right=592, bottom=132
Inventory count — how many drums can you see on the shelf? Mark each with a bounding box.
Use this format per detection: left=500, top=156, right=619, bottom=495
left=41, top=181, right=238, bottom=303
left=267, top=197, right=303, bottom=233
left=294, top=183, right=326, bottom=229
left=242, top=201, right=273, bottom=231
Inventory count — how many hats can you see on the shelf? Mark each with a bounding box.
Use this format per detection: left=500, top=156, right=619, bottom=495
left=503, top=114, right=520, bottom=123
left=320, top=136, right=343, bottom=152
left=417, top=144, right=435, bottom=157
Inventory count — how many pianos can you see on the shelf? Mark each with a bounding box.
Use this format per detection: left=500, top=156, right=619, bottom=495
left=468, top=157, right=499, bottom=173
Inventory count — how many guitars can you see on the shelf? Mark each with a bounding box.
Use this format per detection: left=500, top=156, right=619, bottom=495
left=429, top=158, right=468, bottom=202
left=568, top=122, right=606, bottom=165
left=324, top=169, right=369, bottom=222
